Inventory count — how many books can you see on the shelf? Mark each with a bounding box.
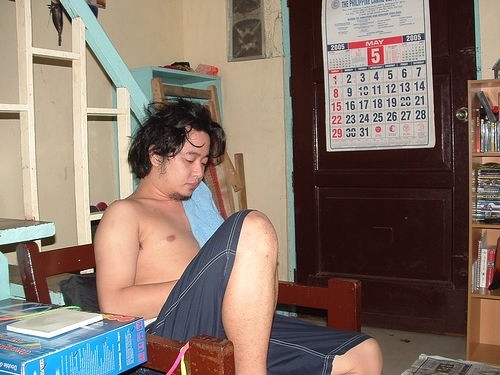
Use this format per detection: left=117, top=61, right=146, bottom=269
left=472, top=109, right=475, bottom=152
left=472, top=233, right=500, bottom=292
left=6, top=310, right=103, bottom=338
left=476, top=92, right=500, bottom=152
left=477, top=164, right=500, bottom=219
left=472, top=172, right=477, bottom=220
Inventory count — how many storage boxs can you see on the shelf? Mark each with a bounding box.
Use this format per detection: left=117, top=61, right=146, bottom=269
left=0, top=297, right=148, bottom=375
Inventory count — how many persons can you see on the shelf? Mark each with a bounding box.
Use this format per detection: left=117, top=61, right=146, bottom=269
left=94, top=99, right=383, bottom=375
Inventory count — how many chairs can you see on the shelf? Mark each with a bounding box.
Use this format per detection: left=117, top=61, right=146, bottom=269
left=151, top=77, right=247, bottom=220
left=16, top=222, right=363, bottom=375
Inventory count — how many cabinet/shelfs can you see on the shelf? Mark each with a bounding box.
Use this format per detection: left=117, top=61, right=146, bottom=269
left=466, top=78, right=500, bottom=366
left=112, top=67, right=223, bottom=198
